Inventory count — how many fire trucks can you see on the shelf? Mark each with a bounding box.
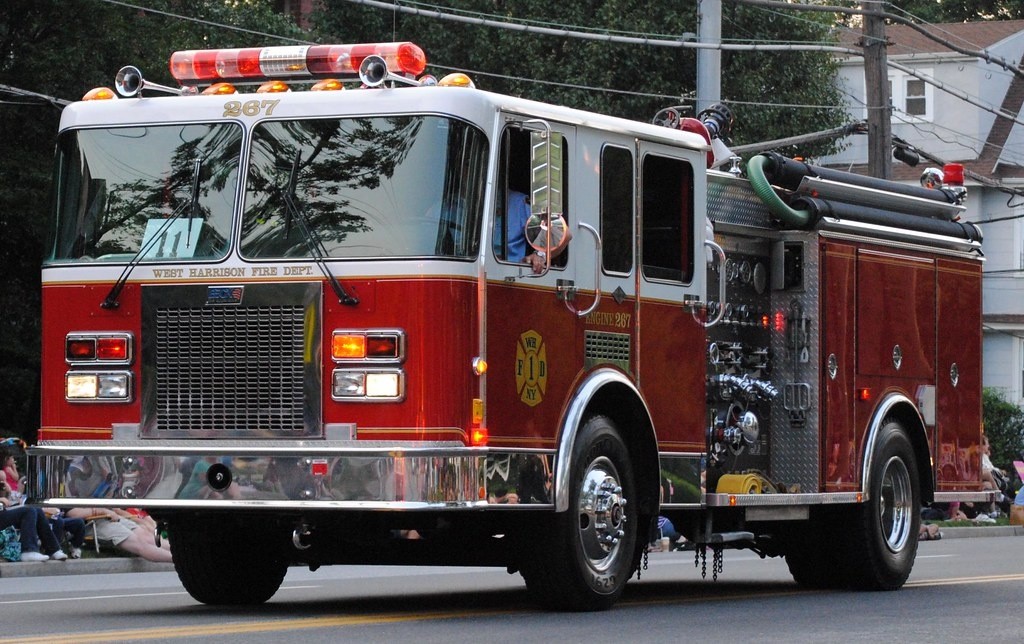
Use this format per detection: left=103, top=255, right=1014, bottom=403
left=26, top=42, right=1007, bottom=611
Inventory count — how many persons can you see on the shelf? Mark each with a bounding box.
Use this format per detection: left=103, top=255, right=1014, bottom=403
left=918, top=436, right=1024, bottom=540
left=420, top=140, right=573, bottom=275
left=0, top=444, right=173, bottom=564
left=650, top=515, right=681, bottom=552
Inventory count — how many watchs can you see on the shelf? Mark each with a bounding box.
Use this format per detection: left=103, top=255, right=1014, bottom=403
left=534, top=250, right=546, bottom=262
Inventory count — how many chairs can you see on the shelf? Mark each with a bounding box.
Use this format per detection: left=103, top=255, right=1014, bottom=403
left=82, top=515, right=139, bottom=554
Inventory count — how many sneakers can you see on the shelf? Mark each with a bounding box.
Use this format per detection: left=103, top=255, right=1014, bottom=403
left=50, top=550, right=68, bottom=560
left=71, top=546, right=82, bottom=558
left=21, top=552, right=50, bottom=562
left=975, top=514, right=996, bottom=524
left=990, top=511, right=997, bottom=518
